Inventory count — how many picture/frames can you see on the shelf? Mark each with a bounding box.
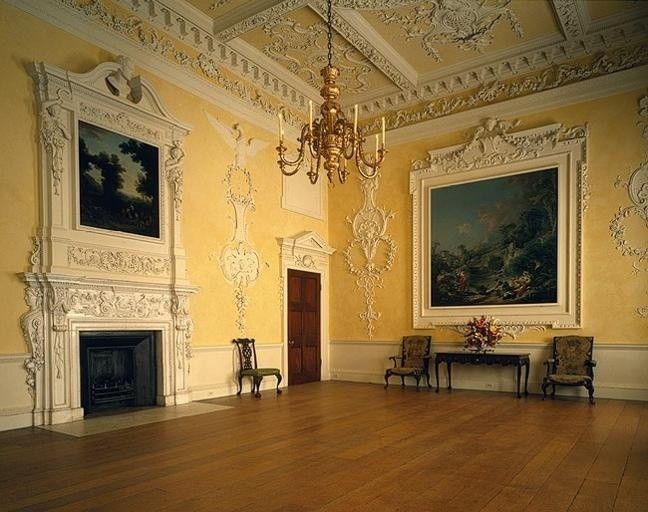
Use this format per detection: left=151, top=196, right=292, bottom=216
left=407, top=115, right=588, bottom=330
left=73, top=116, right=166, bottom=245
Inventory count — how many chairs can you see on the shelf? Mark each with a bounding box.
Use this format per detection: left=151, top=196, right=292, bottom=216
left=542, top=335, right=598, bottom=404
left=232, top=338, right=282, bottom=398
left=384, top=335, right=434, bottom=393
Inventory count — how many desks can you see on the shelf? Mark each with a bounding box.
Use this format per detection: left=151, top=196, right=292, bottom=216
left=434, top=351, right=531, bottom=399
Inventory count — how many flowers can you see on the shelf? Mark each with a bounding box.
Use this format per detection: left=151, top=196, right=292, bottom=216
left=457, top=316, right=516, bottom=352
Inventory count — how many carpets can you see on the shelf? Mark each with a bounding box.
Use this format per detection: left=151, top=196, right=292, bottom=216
left=38, top=401, right=235, bottom=438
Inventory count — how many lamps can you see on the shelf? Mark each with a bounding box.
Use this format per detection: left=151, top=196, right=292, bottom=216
left=275, top=0, right=390, bottom=191
